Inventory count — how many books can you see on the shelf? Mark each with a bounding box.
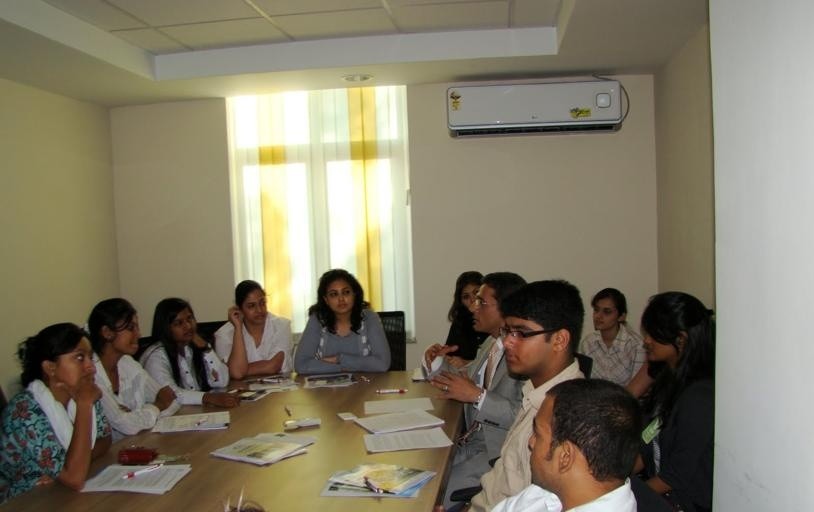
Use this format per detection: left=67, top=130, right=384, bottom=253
left=228, top=375, right=301, bottom=402
left=210, top=432, right=316, bottom=466
left=352, top=397, right=454, bottom=454
left=150, top=411, right=230, bottom=434
left=80, top=462, right=192, bottom=495
left=320, top=460, right=437, bottom=499
left=303, top=372, right=358, bottom=388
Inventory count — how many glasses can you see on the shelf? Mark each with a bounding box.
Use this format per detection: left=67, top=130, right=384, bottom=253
left=499, top=326, right=553, bottom=338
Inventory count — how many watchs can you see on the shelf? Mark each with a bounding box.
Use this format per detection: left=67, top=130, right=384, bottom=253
left=201, top=343, right=212, bottom=353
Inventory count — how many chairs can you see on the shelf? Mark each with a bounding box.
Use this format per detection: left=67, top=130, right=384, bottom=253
left=193, top=321, right=230, bottom=353
left=450, top=351, right=682, bottom=511
left=374, top=311, right=407, bottom=371
left=131, top=336, right=160, bottom=360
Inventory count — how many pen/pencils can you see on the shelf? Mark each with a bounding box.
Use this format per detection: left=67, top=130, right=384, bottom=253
left=257, top=379, right=282, bottom=383
left=365, top=481, right=383, bottom=494
left=376, top=389, right=408, bottom=393
left=285, top=405, right=292, bottom=416
left=195, top=418, right=208, bottom=426
left=123, top=463, right=163, bottom=479
left=221, top=484, right=245, bottom=512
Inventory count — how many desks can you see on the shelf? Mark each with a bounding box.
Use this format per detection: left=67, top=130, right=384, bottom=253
left=1, top=368, right=468, bottom=512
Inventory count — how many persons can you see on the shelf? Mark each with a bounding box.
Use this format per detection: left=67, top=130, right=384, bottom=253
left=294, top=268, right=392, bottom=376
left=139, top=297, right=241, bottom=408
left=212, top=279, right=293, bottom=380
left=423, top=270, right=713, bottom=511
left=0, top=321, right=113, bottom=506
left=80, top=297, right=181, bottom=444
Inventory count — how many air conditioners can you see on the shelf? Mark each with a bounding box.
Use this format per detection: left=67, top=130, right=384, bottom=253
left=446, top=79, right=621, bottom=134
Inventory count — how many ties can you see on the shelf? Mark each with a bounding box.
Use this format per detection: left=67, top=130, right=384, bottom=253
left=484, top=342, right=500, bottom=389
